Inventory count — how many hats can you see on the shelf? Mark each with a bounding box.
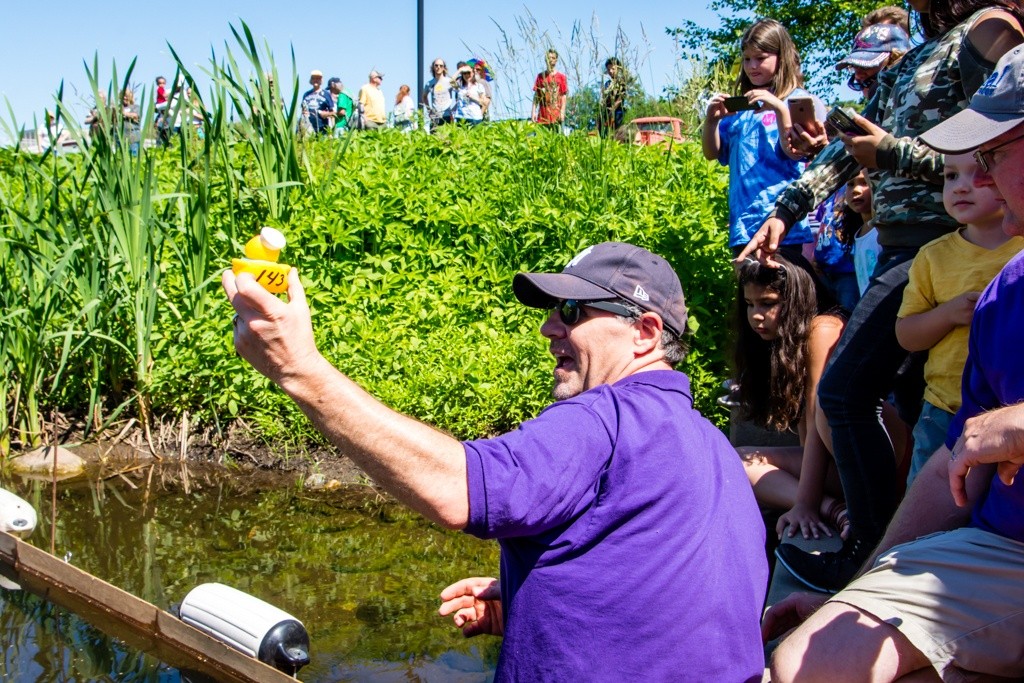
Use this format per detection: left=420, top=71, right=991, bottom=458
left=834, top=22, right=911, bottom=71
left=508, top=241, right=688, bottom=340
left=310, top=70, right=322, bottom=77
left=915, top=41, right=1024, bottom=155
left=370, top=72, right=384, bottom=79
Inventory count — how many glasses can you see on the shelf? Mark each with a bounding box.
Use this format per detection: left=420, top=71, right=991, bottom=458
left=435, top=64, right=443, bottom=68
left=973, top=134, right=1024, bottom=172
left=463, top=71, right=470, bottom=75
left=473, top=68, right=481, bottom=72
left=848, top=72, right=879, bottom=92
left=557, top=295, right=641, bottom=329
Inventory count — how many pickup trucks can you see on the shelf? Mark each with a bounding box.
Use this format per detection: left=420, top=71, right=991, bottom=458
left=631, top=116, right=693, bottom=147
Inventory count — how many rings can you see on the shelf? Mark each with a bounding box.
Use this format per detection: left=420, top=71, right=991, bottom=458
left=951, top=452, right=955, bottom=461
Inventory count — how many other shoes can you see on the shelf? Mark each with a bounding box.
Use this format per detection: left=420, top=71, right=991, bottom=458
left=825, top=499, right=851, bottom=542
left=774, top=530, right=881, bottom=595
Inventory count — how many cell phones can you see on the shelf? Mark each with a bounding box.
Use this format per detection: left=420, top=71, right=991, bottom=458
left=787, top=99, right=817, bottom=139
left=826, top=107, right=868, bottom=137
left=723, top=96, right=761, bottom=113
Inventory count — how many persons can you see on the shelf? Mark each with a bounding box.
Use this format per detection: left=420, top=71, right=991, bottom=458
left=221, top=243, right=768, bottom=682
left=37, top=76, right=205, bottom=157
left=301, top=58, right=494, bottom=138
left=532, top=49, right=568, bottom=133
left=599, top=57, right=626, bottom=139
left=703, top=1, right=1024, bottom=682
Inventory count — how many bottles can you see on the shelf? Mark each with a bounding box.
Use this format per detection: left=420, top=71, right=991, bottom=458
left=244, top=227, right=287, bottom=261
left=178, top=580, right=312, bottom=677
